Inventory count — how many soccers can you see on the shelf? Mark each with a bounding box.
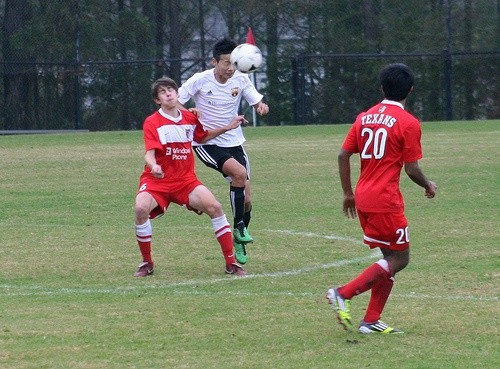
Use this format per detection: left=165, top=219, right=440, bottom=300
left=229, top=43, right=263, bottom=72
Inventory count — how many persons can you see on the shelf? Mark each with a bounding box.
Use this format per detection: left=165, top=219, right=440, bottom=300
left=326, top=63, right=438, bottom=334
left=135, top=77, right=248, bottom=276
left=177, top=38, right=269, bottom=265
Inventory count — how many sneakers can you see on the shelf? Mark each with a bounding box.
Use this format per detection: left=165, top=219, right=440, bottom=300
left=233, top=242, right=248, bottom=264
left=326, top=285, right=354, bottom=331
left=357, top=319, right=404, bottom=336
left=233, top=226, right=253, bottom=244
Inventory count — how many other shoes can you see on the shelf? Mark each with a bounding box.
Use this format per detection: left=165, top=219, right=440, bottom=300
left=224, top=262, right=247, bottom=276
left=134, top=261, right=154, bottom=277
